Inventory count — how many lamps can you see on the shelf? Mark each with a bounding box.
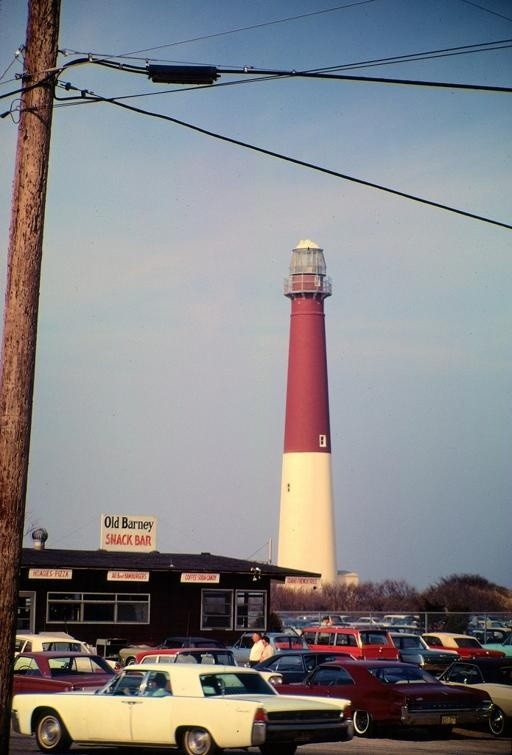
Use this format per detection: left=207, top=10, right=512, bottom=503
left=250, top=567, right=261, bottom=581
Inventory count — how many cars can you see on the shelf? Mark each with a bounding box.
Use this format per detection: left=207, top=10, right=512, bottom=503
left=230, top=632, right=296, bottom=666
left=269, top=661, right=491, bottom=736
left=433, top=660, right=512, bottom=739
left=11, top=650, right=118, bottom=695
left=281, top=613, right=512, bottom=674
left=252, top=650, right=360, bottom=683
left=13, top=633, right=235, bottom=675
left=9, top=665, right=356, bottom=755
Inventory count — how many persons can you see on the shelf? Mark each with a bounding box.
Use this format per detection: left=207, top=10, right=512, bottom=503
left=121, top=672, right=170, bottom=697
left=258, top=635, right=275, bottom=665
left=317, top=615, right=334, bottom=644
left=247, top=631, right=265, bottom=668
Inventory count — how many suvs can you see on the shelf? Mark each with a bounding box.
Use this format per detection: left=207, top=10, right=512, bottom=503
left=278, top=625, right=401, bottom=661
